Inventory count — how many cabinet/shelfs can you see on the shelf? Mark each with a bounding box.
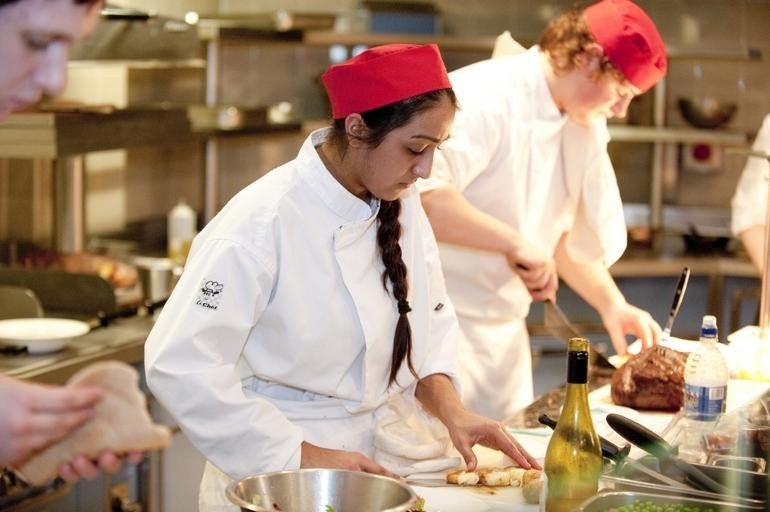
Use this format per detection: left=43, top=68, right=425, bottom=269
left=303, top=31, right=758, bottom=276
left=2, top=29, right=302, bottom=256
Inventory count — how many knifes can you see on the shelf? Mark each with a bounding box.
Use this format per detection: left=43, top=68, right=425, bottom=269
left=661, top=265, right=691, bottom=342
left=395, top=477, right=513, bottom=488
left=538, top=410, right=741, bottom=494
left=518, top=266, right=619, bottom=374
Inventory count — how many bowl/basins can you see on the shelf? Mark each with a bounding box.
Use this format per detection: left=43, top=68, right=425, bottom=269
left=681, top=96, right=737, bottom=129
left=224, top=467, right=418, bottom=512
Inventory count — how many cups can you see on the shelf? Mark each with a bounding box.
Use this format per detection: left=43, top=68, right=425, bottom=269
left=135, top=263, right=172, bottom=306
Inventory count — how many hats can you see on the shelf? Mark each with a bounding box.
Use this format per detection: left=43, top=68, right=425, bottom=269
left=321, top=43, right=453, bottom=118
left=584, top=0, right=668, bottom=94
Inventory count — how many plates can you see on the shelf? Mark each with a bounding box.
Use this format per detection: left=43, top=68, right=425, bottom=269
left=0, top=317, right=88, bottom=354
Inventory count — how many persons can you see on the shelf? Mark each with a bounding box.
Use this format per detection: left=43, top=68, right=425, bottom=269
left=1, top=0, right=150, bottom=511
left=407, top=1, right=668, bottom=435
left=141, top=43, right=545, bottom=512
left=728, top=114, right=770, bottom=342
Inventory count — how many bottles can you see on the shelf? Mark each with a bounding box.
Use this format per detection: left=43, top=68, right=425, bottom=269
left=542, top=336, right=599, bottom=512
left=684, top=316, right=726, bottom=448
left=166, top=196, right=197, bottom=261
